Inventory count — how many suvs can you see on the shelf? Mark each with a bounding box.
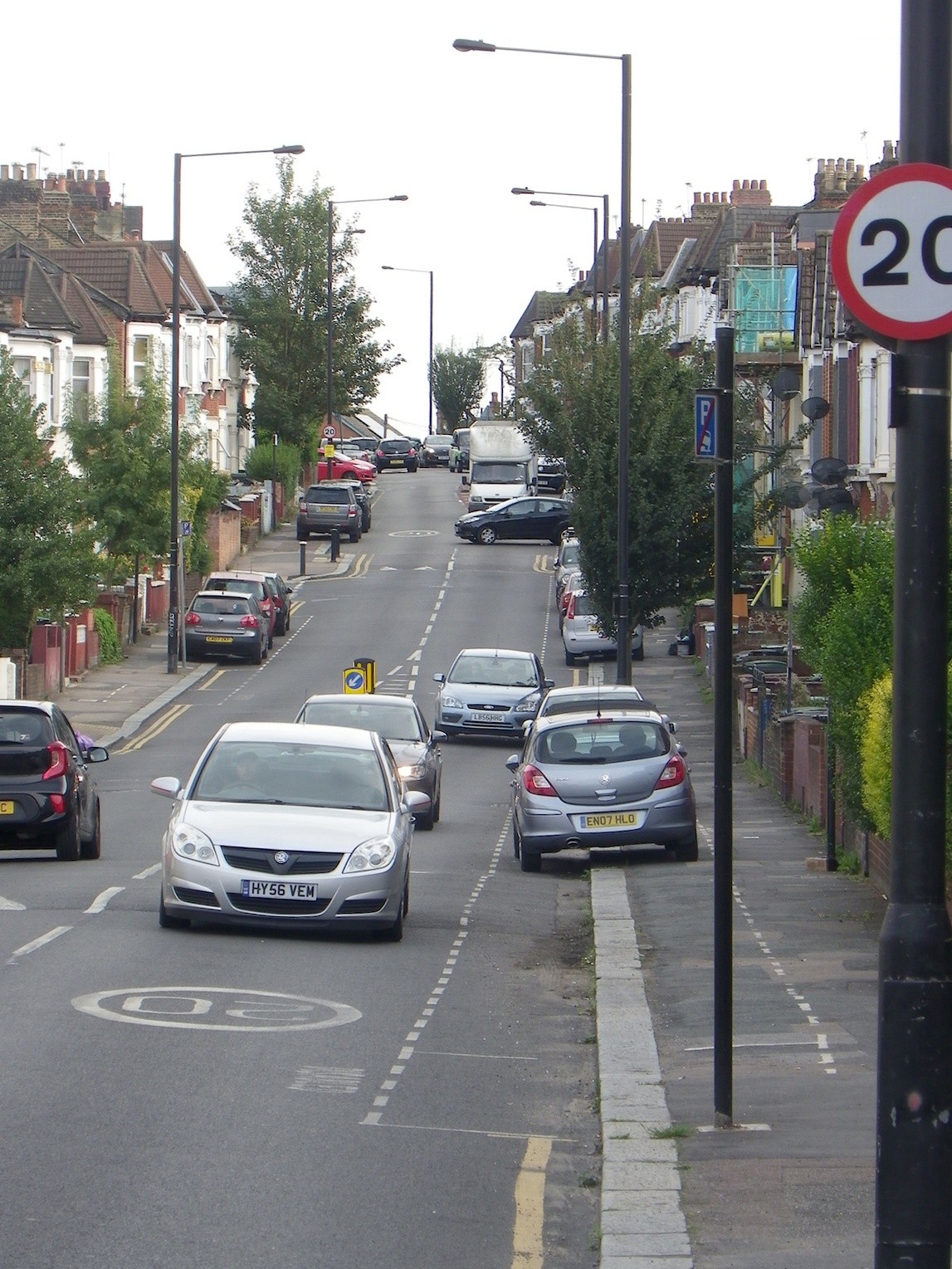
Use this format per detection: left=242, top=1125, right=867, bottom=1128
left=318, top=479, right=372, bottom=534
left=296, top=484, right=364, bottom=543
left=191, top=572, right=284, bottom=652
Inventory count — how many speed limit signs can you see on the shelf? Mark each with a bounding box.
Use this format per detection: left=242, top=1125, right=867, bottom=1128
left=829, top=161, right=952, bottom=339
left=323, top=425, right=336, bottom=439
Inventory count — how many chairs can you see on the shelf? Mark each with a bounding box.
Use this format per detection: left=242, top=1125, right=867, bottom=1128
left=550, top=733, right=583, bottom=759
left=201, top=602, right=214, bottom=612
left=233, top=603, right=248, bottom=613
left=613, top=726, right=652, bottom=757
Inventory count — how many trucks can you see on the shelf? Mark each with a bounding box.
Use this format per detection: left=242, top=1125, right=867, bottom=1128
left=461, top=420, right=538, bottom=513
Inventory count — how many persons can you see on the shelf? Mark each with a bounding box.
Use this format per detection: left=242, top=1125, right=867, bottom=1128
left=223, top=751, right=271, bottom=792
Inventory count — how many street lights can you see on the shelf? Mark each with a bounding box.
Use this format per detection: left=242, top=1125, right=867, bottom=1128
left=167, top=145, right=305, bottom=674
left=326, top=194, right=411, bottom=480
left=381, top=265, right=433, bottom=435
left=452, top=37, right=631, bottom=687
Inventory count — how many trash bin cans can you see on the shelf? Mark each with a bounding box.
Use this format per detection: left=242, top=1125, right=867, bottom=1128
left=677, top=640, right=691, bottom=656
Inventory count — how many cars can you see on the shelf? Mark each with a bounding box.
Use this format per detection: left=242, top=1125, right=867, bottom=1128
left=454, top=496, right=580, bottom=546
left=0, top=698, right=110, bottom=861
left=536, top=449, right=568, bottom=495
left=148, top=720, right=433, bottom=945
left=432, top=648, right=556, bottom=740
left=560, top=588, right=646, bottom=667
left=552, top=537, right=601, bottom=637
left=226, top=569, right=293, bottom=631
left=177, top=590, right=272, bottom=667
left=292, top=694, right=449, bottom=832
left=505, top=709, right=700, bottom=873
left=316, top=448, right=378, bottom=486
left=520, top=684, right=672, bottom=769
left=320, top=428, right=471, bottom=473
